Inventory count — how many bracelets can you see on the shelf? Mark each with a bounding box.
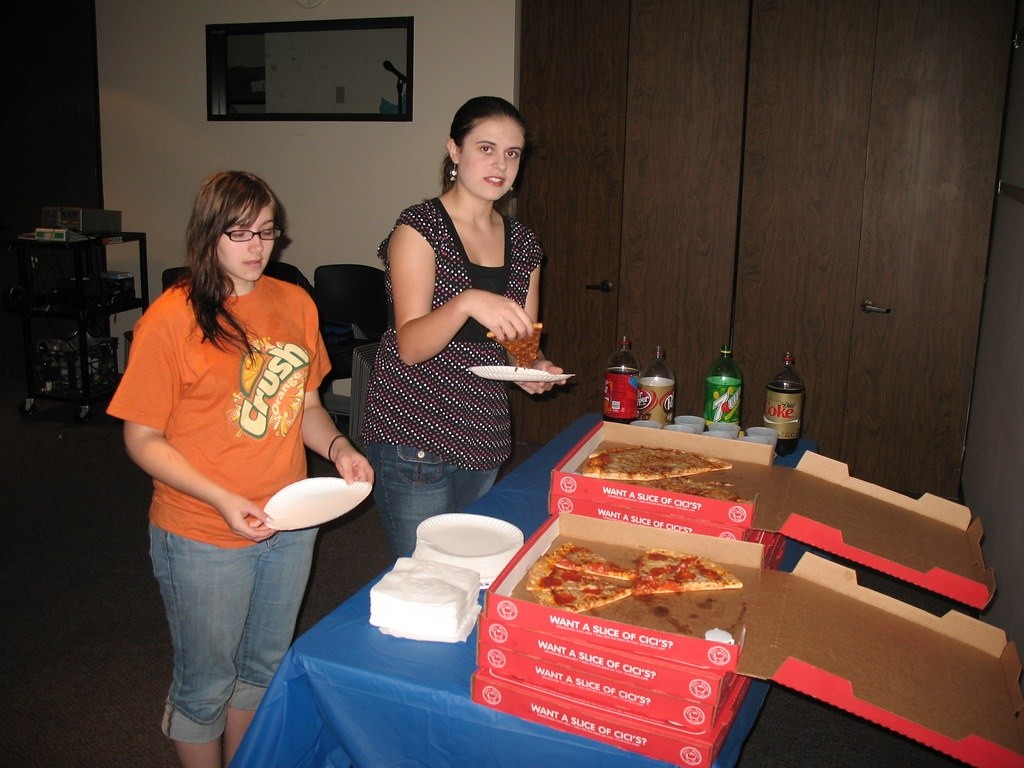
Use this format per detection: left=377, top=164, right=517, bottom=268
left=329, top=435, right=347, bottom=461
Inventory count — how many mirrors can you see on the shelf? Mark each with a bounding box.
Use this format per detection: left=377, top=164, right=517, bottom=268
left=206, top=16, right=415, bottom=122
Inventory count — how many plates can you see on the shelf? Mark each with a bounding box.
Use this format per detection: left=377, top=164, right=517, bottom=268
left=262, top=477, right=372, bottom=531
left=470, top=365, right=575, bottom=381
left=412, top=513, right=524, bottom=584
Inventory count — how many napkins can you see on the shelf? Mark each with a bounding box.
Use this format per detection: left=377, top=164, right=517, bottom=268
left=368, top=557, right=482, bottom=643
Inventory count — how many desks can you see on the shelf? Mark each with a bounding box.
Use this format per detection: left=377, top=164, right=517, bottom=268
left=213, top=411, right=818, bottom=768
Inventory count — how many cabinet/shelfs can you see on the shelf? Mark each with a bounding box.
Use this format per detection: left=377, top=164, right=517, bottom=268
left=501, top=1, right=1015, bottom=503
left=16, top=232, right=150, bottom=423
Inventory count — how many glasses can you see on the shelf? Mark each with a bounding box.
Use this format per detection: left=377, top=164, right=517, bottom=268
left=222, top=224, right=281, bottom=242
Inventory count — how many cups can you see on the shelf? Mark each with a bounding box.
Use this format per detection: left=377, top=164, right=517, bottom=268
left=664, top=425, right=696, bottom=434
left=738, top=436, right=769, bottom=445
left=674, top=416, right=706, bottom=434
left=631, top=421, right=662, bottom=429
left=701, top=431, right=733, bottom=439
left=708, top=423, right=741, bottom=439
left=746, top=427, right=778, bottom=451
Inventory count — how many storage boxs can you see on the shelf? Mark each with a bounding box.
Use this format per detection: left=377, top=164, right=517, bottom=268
left=471, top=420, right=1024, bottom=768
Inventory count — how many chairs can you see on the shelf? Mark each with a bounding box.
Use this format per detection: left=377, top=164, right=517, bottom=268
left=124, top=263, right=390, bottom=442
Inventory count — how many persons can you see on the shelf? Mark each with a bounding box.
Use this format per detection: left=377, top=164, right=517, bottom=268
left=107, top=171, right=375, bottom=768
left=363, top=96, right=566, bottom=567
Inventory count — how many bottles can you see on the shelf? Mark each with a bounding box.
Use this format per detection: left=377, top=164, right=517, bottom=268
left=705, top=346, right=742, bottom=431
left=638, top=346, right=675, bottom=428
left=764, top=353, right=805, bottom=456
left=603, top=337, right=639, bottom=425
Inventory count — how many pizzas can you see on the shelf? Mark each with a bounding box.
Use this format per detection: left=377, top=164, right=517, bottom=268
left=524, top=541, right=636, bottom=614
left=631, top=548, right=743, bottom=595
left=487, top=322, right=543, bottom=369
left=582, top=446, right=733, bottom=479
